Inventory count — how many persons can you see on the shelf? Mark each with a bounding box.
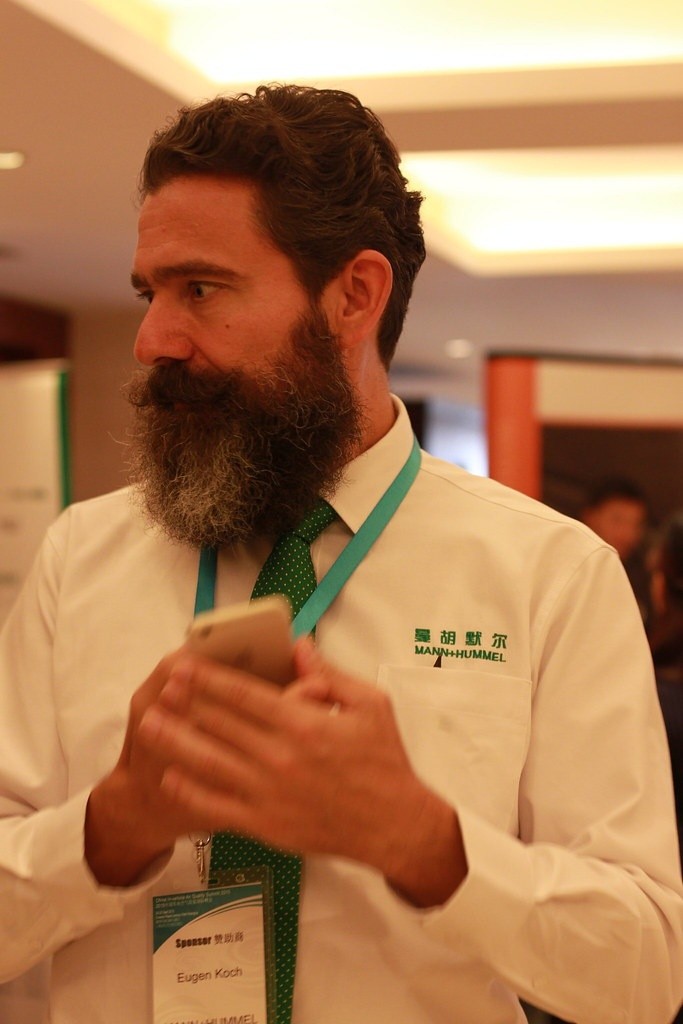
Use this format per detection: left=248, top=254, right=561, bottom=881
left=0, top=84, right=683, bottom=1024
left=578, top=475, right=682, bottom=866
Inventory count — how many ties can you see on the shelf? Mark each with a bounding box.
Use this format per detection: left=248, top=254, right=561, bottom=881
left=206, top=494, right=340, bottom=1024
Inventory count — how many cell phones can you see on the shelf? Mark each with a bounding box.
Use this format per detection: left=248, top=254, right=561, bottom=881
left=185, top=595, right=301, bottom=694
